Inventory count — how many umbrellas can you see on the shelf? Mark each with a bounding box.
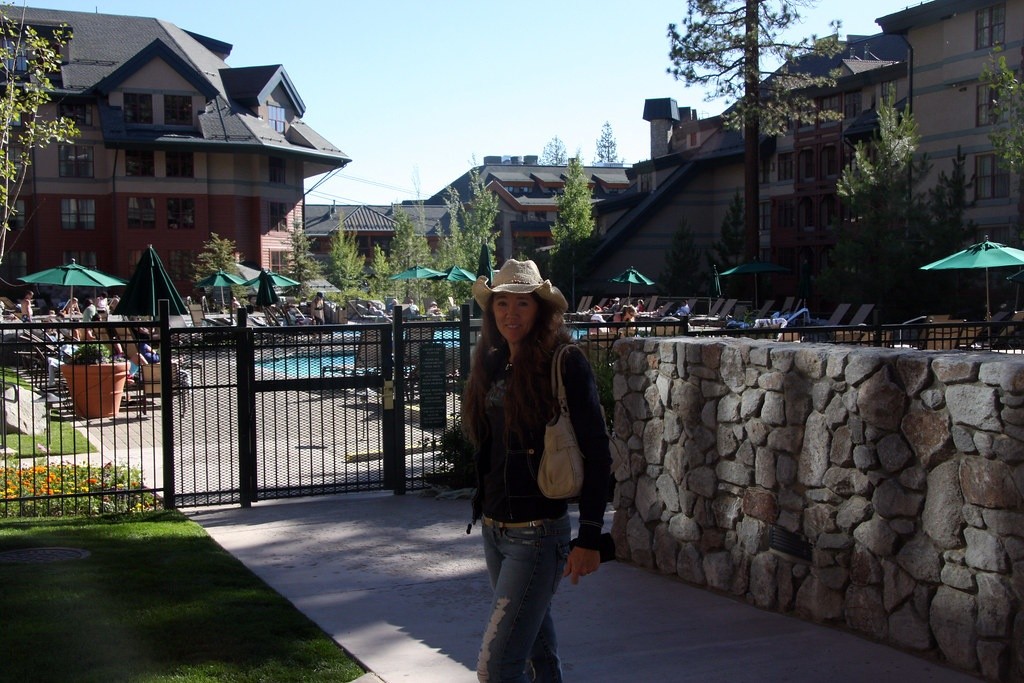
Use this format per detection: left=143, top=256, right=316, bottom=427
left=473, top=238, right=493, bottom=318
left=194, top=268, right=245, bottom=305
left=1006, top=270, right=1024, bottom=282
left=610, top=266, right=656, bottom=296
left=256, top=268, right=281, bottom=307
left=718, top=256, right=793, bottom=309
left=388, top=263, right=448, bottom=315
left=112, top=244, right=189, bottom=321
left=243, top=267, right=300, bottom=288
left=919, top=235, right=1024, bottom=347
left=17, top=259, right=130, bottom=320
left=427, top=265, right=477, bottom=297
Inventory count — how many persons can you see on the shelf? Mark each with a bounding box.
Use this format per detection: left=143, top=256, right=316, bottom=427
left=428, top=301, right=443, bottom=318
left=367, top=303, right=392, bottom=322
left=590, top=297, right=690, bottom=332
left=232, top=297, right=240, bottom=308
left=310, top=292, right=324, bottom=325
left=407, top=298, right=419, bottom=314
left=463, top=260, right=614, bottom=682
left=388, top=298, right=398, bottom=312
left=21, top=290, right=34, bottom=322
left=44, top=292, right=159, bottom=385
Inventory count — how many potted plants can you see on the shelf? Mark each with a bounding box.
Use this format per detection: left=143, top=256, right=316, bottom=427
left=61, top=342, right=130, bottom=419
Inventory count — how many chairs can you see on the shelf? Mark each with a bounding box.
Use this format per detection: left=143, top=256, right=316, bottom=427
left=1, top=297, right=1024, bottom=422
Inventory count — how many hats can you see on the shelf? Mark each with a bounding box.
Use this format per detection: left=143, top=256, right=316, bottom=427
left=472, top=258, right=568, bottom=316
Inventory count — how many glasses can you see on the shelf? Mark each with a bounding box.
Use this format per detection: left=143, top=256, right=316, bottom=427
left=493, top=363, right=513, bottom=409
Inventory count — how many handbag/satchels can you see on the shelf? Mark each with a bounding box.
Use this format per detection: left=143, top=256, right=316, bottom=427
left=537, top=343, right=624, bottom=499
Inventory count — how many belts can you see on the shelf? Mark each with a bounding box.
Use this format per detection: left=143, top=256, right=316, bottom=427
left=482, top=514, right=568, bottom=528
